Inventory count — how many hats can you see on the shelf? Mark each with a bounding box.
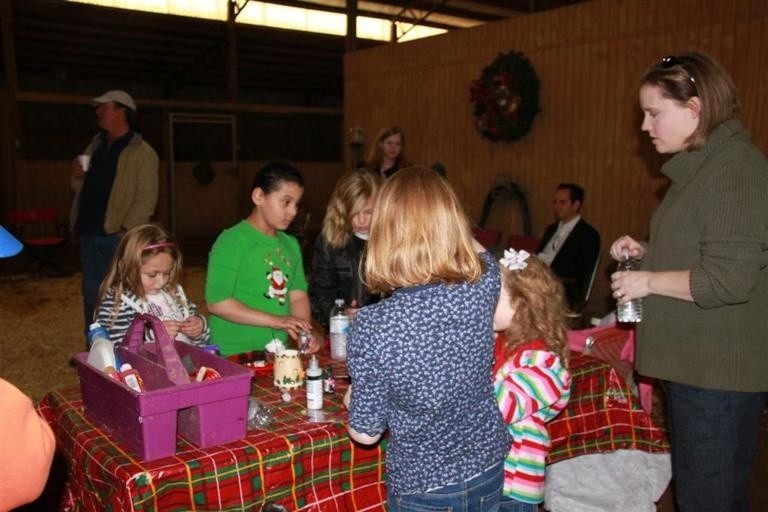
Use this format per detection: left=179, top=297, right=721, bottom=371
left=0, top=227, right=24, bottom=261
left=94, top=91, right=136, bottom=110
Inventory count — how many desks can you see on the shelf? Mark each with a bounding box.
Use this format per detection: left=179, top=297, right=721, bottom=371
left=35, top=338, right=674, bottom=512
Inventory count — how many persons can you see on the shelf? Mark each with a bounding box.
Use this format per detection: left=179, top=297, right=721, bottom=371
left=532, top=181, right=600, bottom=307
left=263, top=268, right=288, bottom=305
left=493, top=250, right=573, bottom=512
left=70, top=90, right=160, bottom=366
left=1, top=378, right=68, bottom=511
left=202, top=162, right=322, bottom=359
left=343, top=164, right=515, bottom=511
left=364, top=126, right=404, bottom=177
left=610, top=50, right=767, bottom=511
left=308, top=168, right=401, bottom=334
left=91, top=223, right=210, bottom=346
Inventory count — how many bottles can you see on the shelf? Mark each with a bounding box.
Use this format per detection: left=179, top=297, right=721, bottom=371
left=613, top=249, right=642, bottom=324
left=326, top=299, right=349, bottom=362
left=297, top=332, right=310, bottom=356
left=306, top=353, right=323, bottom=409
left=324, top=367, right=335, bottom=393
left=106, top=366, right=124, bottom=384
left=117, top=362, right=146, bottom=395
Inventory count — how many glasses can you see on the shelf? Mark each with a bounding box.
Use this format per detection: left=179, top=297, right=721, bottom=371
left=661, top=55, right=696, bottom=84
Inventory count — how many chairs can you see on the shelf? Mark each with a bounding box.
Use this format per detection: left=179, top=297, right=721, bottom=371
left=8, top=206, right=64, bottom=277
left=565, top=245, right=602, bottom=328
left=566, top=320, right=653, bottom=416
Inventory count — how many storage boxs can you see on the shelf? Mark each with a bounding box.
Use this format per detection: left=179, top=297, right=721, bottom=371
left=70, top=313, right=254, bottom=463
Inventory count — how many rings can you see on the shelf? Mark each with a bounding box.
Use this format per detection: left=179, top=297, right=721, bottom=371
left=615, top=291, right=623, bottom=297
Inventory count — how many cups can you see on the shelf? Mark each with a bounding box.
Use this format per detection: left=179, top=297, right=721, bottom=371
left=79, top=155, right=90, bottom=172
left=272, top=349, right=302, bottom=389
